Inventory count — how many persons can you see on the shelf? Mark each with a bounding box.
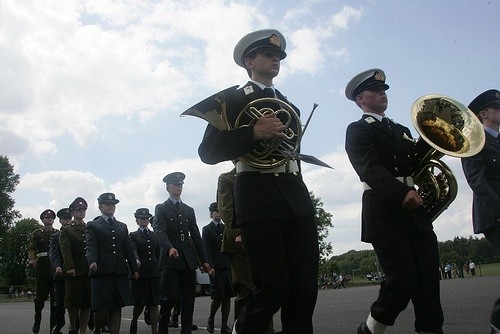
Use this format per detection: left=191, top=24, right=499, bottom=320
left=464, top=259, right=477, bottom=277
left=128, top=208, right=198, bottom=334
left=29, top=210, right=62, bottom=334
left=48, top=208, right=80, bottom=333
left=345, top=68, right=449, bottom=334
left=198, top=29, right=320, bottom=334
left=460, top=88, right=500, bottom=334
left=87, top=301, right=111, bottom=333
left=440, top=259, right=460, bottom=280
left=85, top=193, right=141, bottom=334
left=200, top=202, right=233, bottom=334
left=9, top=285, right=32, bottom=299
left=58, top=201, right=91, bottom=334
left=154, top=172, right=212, bottom=334
left=320, top=270, right=351, bottom=290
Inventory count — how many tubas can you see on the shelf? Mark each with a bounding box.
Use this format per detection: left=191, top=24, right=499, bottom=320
left=403, top=94, right=485, bottom=223
left=180, top=84, right=319, bottom=177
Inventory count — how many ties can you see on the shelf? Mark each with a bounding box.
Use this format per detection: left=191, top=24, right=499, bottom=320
left=176, top=201, right=181, bottom=212
left=48, top=230, right=51, bottom=235
left=382, top=118, right=392, bottom=134
left=108, top=218, right=113, bottom=227
left=79, top=223, right=83, bottom=228
left=143, top=229, right=148, bottom=236
left=264, top=86, right=274, bottom=104
left=217, top=223, right=220, bottom=230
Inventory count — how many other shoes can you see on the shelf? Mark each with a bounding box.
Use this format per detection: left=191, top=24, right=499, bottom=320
left=357, top=322, right=373, bottom=334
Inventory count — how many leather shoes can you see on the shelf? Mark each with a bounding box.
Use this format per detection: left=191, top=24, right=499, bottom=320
left=32, top=313, right=232, bottom=334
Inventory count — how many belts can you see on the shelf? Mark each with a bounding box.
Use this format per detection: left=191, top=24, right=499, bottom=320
left=235, top=159, right=299, bottom=174
left=363, top=177, right=413, bottom=190
left=168, top=233, right=188, bottom=241
left=36, top=252, right=48, bottom=257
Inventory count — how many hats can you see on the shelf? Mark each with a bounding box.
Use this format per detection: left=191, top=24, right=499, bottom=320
left=468, top=90, right=500, bottom=114
left=40, top=209, right=57, bottom=220
left=209, top=202, right=218, bottom=210
left=68, top=197, right=88, bottom=211
left=233, top=28, right=287, bottom=68
left=98, top=193, right=119, bottom=204
left=135, top=208, right=152, bottom=218
left=347, top=69, right=390, bottom=101
left=57, top=208, right=73, bottom=219
left=163, top=172, right=185, bottom=184
